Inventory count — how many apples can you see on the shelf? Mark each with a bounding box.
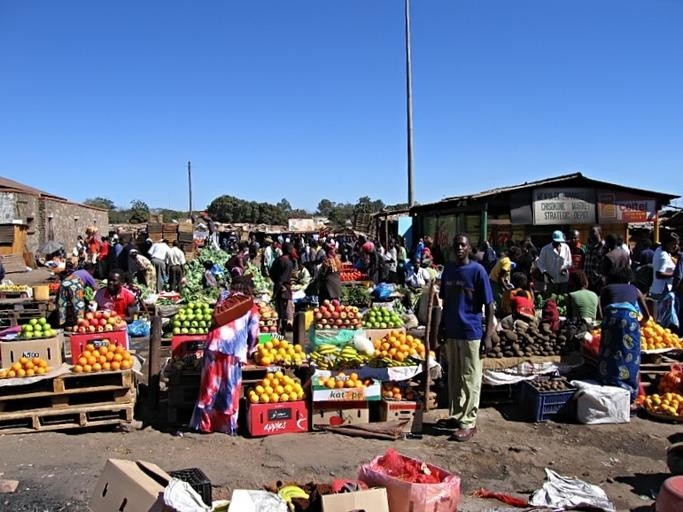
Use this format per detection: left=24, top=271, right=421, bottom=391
left=256, top=302, right=278, bottom=334
left=313, top=299, right=404, bottom=330
left=73, top=310, right=122, bottom=333
left=640, top=320, right=682, bottom=350
left=173, top=302, right=214, bottom=334
left=17, top=317, right=56, bottom=338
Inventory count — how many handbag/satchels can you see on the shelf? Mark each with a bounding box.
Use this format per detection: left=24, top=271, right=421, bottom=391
left=212, top=294, right=255, bottom=328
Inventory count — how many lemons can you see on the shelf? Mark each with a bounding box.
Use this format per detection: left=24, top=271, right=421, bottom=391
left=247, top=370, right=307, bottom=404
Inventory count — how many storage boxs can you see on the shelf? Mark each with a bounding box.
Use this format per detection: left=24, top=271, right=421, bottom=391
left=365, top=328, right=406, bottom=350
left=0, top=334, right=65, bottom=369
left=358, top=449, right=461, bottom=512
left=85, top=459, right=391, bottom=512
left=32, top=286, right=49, bottom=301
left=380, top=398, right=423, bottom=433
left=311, top=379, right=383, bottom=400
left=254, top=332, right=279, bottom=346
left=241, top=400, right=308, bottom=437
left=313, top=403, right=365, bottom=432
left=68, top=331, right=128, bottom=367
left=147, top=221, right=196, bottom=262
left=169, top=335, right=210, bottom=371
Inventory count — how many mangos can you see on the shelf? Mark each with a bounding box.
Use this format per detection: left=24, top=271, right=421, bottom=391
left=644, top=391, right=683, bottom=416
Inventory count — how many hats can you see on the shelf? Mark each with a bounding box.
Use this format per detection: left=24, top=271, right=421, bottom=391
left=552, top=230, right=566, bottom=243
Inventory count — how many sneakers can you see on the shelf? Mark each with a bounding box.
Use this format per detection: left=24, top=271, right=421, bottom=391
left=436, top=416, right=465, bottom=429
left=452, top=424, right=478, bottom=442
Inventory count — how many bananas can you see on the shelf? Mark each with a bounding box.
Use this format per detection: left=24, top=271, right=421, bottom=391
left=278, top=485, right=309, bottom=502
left=310, top=345, right=369, bottom=370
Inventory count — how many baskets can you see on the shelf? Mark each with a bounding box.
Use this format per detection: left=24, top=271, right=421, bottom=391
left=518, top=379, right=580, bottom=423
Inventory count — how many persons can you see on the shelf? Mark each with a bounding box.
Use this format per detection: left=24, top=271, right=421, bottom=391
left=596, top=265, right=651, bottom=414
left=432, top=231, right=498, bottom=444
left=187, top=274, right=261, bottom=435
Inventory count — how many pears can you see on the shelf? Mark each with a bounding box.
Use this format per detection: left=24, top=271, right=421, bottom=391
left=373, top=331, right=435, bottom=362
left=256, top=338, right=306, bottom=366
